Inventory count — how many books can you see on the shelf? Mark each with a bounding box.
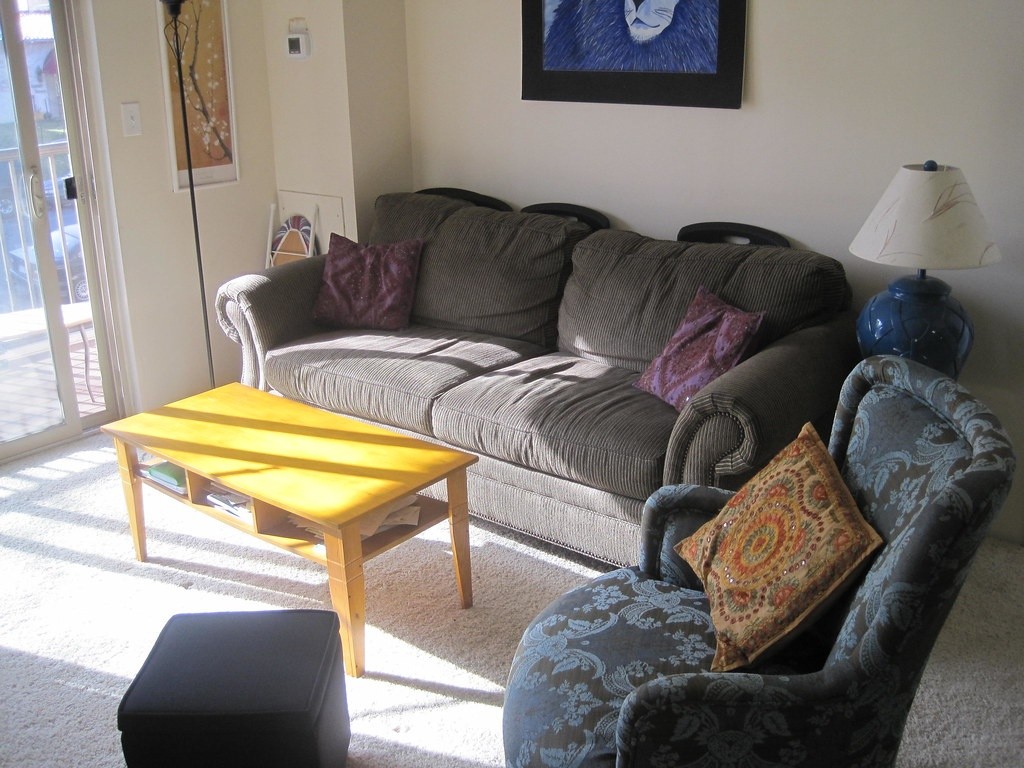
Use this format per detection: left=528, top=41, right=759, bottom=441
left=202, top=481, right=253, bottom=527
left=139, top=460, right=187, bottom=494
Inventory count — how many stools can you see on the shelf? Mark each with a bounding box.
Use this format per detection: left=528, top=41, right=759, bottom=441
left=117, top=609, right=350, bottom=768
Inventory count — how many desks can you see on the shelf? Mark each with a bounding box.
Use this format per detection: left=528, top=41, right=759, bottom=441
left=0, top=302, right=97, bottom=403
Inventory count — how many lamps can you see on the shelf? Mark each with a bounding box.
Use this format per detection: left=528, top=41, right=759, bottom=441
left=848, top=159, right=1003, bottom=379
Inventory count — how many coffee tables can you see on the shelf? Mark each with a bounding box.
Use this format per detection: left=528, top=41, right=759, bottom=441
left=99, top=381, right=481, bottom=680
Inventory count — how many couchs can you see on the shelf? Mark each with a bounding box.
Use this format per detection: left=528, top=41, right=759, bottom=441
left=214, top=188, right=857, bottom=575
left=501, top=356, right=1017, bottom=768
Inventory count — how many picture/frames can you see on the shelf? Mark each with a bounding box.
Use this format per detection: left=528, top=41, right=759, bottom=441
left=155, top=0, right=241, bottom=194
left=520, top=0, right=746, bottom=109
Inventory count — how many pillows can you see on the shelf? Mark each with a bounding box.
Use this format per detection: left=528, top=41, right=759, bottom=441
left=672, top=422, right=884, bottom=674
left=310, top=233, right=425, bottom=333
left=632, top=284, right=767, bottom=412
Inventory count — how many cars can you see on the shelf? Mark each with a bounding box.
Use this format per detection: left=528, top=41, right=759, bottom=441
left=43, top=173, right=73, bottom=206
left=8, top=224, right=88, bottom=302
